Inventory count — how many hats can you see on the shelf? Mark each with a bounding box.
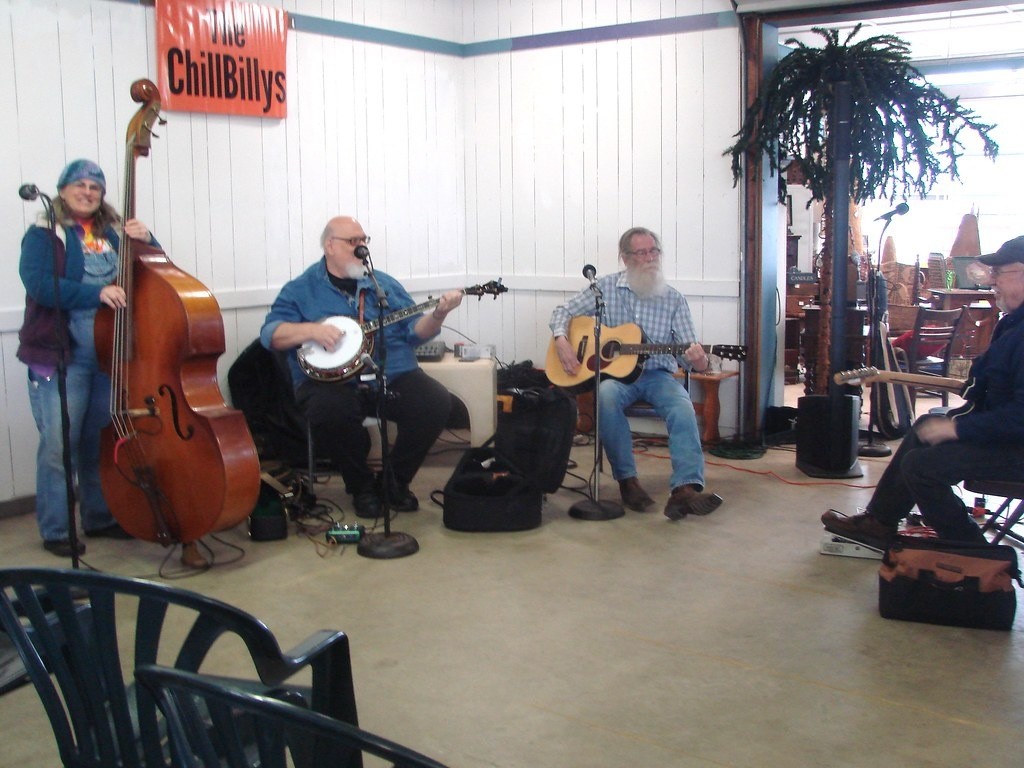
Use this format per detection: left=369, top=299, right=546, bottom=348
left=975, top=235, right=1024, bottom=266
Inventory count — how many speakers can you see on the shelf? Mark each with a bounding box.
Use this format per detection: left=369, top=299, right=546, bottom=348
left=796, top=394, right=861, bottom=474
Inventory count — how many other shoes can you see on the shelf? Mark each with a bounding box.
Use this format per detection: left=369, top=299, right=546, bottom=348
left=85, top=522, right=135, bottom=540
left=43, top=539, right=86, bottom=557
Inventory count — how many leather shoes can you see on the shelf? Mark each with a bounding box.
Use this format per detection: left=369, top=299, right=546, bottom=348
left=352, top=468, right=419, bottom=519
left=663, top=484, right=724, bottom=520
left=821, top=509, right=898, bottom=552
left=619, top=477, right=654, bottom=513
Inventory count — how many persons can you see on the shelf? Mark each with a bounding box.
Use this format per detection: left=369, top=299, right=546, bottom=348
left=17, top=159, right=162, bottom=557
left=821, top=234, right=1024, bottom=550
left=549, top=228, right=724, bottom=521
left=260, top=217, right=463, bottom=515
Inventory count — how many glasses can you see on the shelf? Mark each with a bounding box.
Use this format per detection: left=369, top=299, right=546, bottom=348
left=625, top=248, right=661, bottom=258
left=331, top=236, right=371, bottom=246
left=987, top=269, right=1018, bottom=278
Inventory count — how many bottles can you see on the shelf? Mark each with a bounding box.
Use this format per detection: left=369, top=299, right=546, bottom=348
left=326, top=525, right=373, bottom=541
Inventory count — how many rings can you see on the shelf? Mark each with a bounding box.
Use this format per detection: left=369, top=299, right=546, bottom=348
left=694, top=349, right=698, bottom=353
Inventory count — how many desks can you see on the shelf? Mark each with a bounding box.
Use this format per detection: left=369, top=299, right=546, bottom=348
left=572, top=368, right=742, bottom=443
left=801, top=304, right=869, bottom=377
left=928, top=286, right=1001, bottom=361
left=368, top=351, right=498, bottom=464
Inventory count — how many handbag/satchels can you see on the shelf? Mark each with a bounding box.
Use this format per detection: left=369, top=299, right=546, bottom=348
left=430, top=425, right=543, bottom=532
left=878, top=531, right=1023, bottom=632
left=867, top=268, right=914, bottom=441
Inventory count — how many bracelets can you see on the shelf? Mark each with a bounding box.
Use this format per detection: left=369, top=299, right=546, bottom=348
left=432, top=311, right=447, bottom=321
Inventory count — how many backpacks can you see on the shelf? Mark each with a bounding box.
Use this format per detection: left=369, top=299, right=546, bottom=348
left=511, top=386, right=577, bottom=493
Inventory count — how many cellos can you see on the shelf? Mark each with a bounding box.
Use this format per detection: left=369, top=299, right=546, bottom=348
left=92, top=78, right=261, bottom=548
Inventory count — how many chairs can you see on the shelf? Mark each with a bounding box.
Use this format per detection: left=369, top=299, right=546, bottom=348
left=595, top=362, right=691, bottom=474
left=887, top=305, right=968, bottom=416
left=0, top=568, right=451, bottom=768
left=270, top=357, right=388, bottom=498
left=962, top=477, right=1023, bottom=549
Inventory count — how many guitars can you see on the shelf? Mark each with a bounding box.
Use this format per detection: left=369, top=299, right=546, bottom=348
left=544, top=314, right=748, bottom=394
left=296, top=277, right=508, bottom=382
left=833, top=365, right=965, bottom=396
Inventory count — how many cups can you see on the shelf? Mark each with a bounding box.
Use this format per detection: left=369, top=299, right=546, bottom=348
left=945, top=270, right=953, bottom=290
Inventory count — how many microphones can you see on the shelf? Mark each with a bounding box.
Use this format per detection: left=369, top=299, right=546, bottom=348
left=582, top=264, right=597, bottom=285
left=354, top=245, right=370, bottom=259
left=874, top=203, right=910, bottom=222
left=19, top=184, right=39, bottom=200
